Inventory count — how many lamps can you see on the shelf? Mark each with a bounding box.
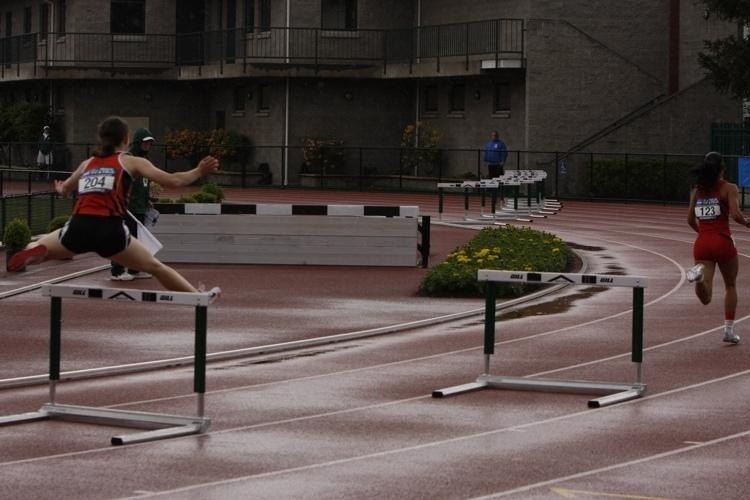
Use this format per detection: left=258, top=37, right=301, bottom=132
left=560, top=159, right=568, bottom=175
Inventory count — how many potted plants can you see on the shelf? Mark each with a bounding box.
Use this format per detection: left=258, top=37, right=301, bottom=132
left=185, top=183, right=225, bottom=214
left=3, top=216, right=33, bottom=271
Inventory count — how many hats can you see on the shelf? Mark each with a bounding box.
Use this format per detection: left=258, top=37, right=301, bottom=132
left=142, top=137, right=156, bottom=142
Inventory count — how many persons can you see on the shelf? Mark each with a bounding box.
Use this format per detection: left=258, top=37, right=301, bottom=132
left=483, top=131, right=506, bottom=207
left=111, top=126, right=155, bottom=282
left=688, top=151, right=750, bottom=343
left=7, top=114, right=221, bottom=307
left=36, top=126, right=54, bottom=180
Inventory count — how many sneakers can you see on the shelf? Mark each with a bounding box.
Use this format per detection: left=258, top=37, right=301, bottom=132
left=695, top=281, right=712, bottom=305
left=111, top=272, right=135, bottom=282
left=207, top=287, right=221, bottom=304
left=7, top=243, right=48, bottom=272
left=722, top=333, right=740, bottom=343
left=128, top=272, right=153, bottom=280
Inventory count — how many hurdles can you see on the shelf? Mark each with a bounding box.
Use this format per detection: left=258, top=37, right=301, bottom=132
left=0, top=284, right=222, bottom=446
left=432, top=268, right=647, bottom=408
left=436, top=170, right=563, bottom=226
left=149, top=203, right=430, bottom=270
